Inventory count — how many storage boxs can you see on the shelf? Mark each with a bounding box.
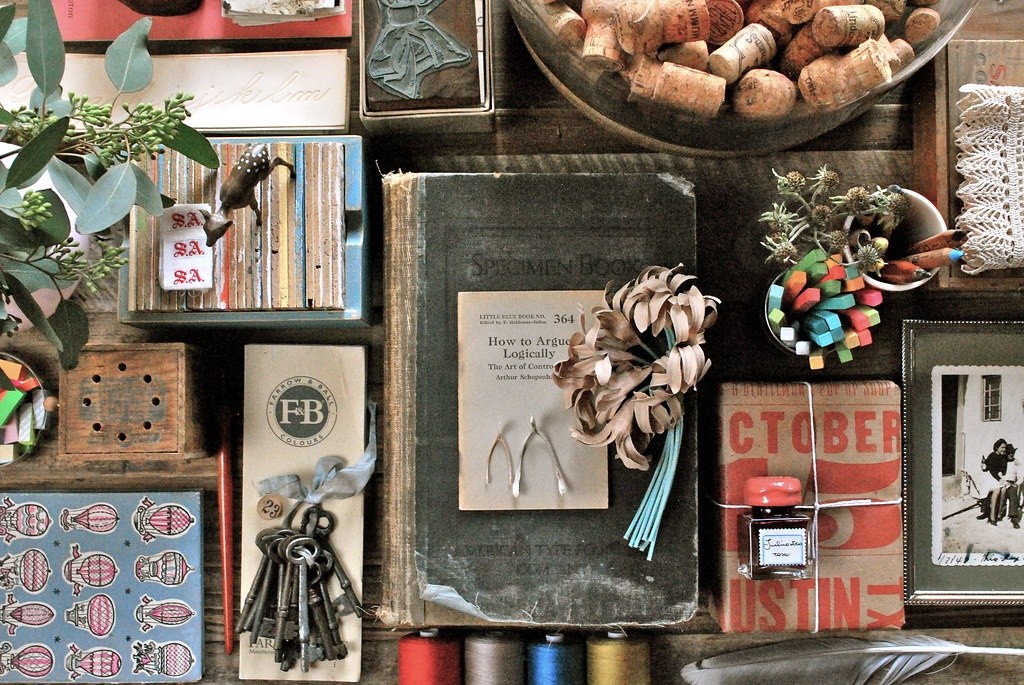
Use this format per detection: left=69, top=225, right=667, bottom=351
left=359, top=0, right=495, bottom=138
left=936, top=39, right=1024, bottom=298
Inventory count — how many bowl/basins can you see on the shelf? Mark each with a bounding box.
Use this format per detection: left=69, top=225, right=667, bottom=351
left=511, top=0, right=979, bottom=158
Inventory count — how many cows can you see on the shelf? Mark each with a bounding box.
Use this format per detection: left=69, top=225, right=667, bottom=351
left=199, top=143, right=296, bottom=247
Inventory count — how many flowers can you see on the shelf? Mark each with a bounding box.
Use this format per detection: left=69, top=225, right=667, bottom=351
left=552, top=263, right=722, bottom=562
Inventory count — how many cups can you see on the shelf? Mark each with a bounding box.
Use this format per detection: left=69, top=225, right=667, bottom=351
left=844, top=187, right=947, bottom=292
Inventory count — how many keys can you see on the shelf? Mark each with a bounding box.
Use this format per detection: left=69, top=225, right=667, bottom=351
left=235, top=500, right=364, bottom=674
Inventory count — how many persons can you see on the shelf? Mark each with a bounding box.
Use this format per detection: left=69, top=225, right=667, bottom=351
left=976, top=439, right=1008, bottom=523
left=994, top=444, right=1024, bottom=529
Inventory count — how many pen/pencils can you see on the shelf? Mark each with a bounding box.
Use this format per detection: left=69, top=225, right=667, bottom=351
left=217, top=402, right=234, bottom=656
left=872, top=229, right=972, bottom=283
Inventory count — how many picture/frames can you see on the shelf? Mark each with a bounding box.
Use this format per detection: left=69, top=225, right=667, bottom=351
left=902, top=319, right=1024, bottom=607
